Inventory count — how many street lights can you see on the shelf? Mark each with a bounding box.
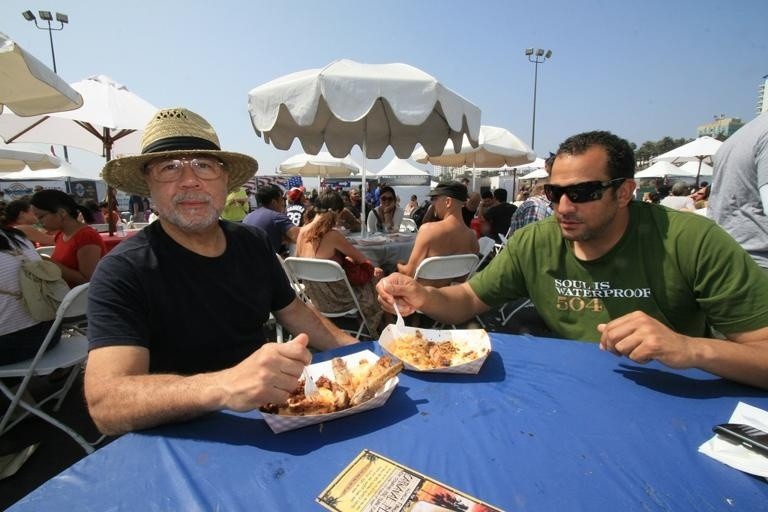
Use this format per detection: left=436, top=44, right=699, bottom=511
left=524, top=47, right=553, bottom=150
left=21, top=9, right=69, bottom=72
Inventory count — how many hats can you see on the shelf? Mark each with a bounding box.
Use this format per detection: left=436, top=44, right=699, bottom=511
left=426, top=182, right=469, bottom=201
left=102, top=108, right=258, bottom=197
left=289, top=186, right=305, bottom=201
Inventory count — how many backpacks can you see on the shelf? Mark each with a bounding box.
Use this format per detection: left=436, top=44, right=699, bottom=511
left=0, top=248, right=70, bottom=323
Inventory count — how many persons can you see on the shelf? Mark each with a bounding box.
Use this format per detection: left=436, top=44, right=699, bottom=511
left=644, top=180, right=711, bottom=217
left=706, top=108, right=768, bottom=269
left=0, top=188, right=151, bottom=364
left=82, top=108, right=361, bottom=436
left=375, top=131, right=768, bottom=384
left=222, top=185, right=555, bottom=329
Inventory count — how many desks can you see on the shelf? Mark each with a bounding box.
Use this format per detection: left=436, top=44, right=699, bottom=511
left=90, top=209, right=151, bottom=255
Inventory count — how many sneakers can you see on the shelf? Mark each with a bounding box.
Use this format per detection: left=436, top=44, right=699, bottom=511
left=0, top=444, right=36, bottom=480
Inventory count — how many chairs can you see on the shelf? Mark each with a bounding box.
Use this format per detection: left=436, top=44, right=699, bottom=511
left=493, top=232, right=531, bottom=327
left=273, top=249, right=374, bottom=341
left=433, top=237, right=495, bottom=330
left=0, top=279, right=108, bottom=456
left=389, top=250, right=480, bottom=329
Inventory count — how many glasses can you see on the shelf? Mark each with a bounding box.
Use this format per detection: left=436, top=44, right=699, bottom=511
left=380, top=196, right=396, bottom=202
left=543, top=178, right=624, bottom=203
left=146, top=159, right=230, bottom=182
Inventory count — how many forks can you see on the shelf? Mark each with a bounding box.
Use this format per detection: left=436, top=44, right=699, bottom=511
left=299, top=368, right=324, bottom=403
left=389, top=300, right=409, bottom=338
